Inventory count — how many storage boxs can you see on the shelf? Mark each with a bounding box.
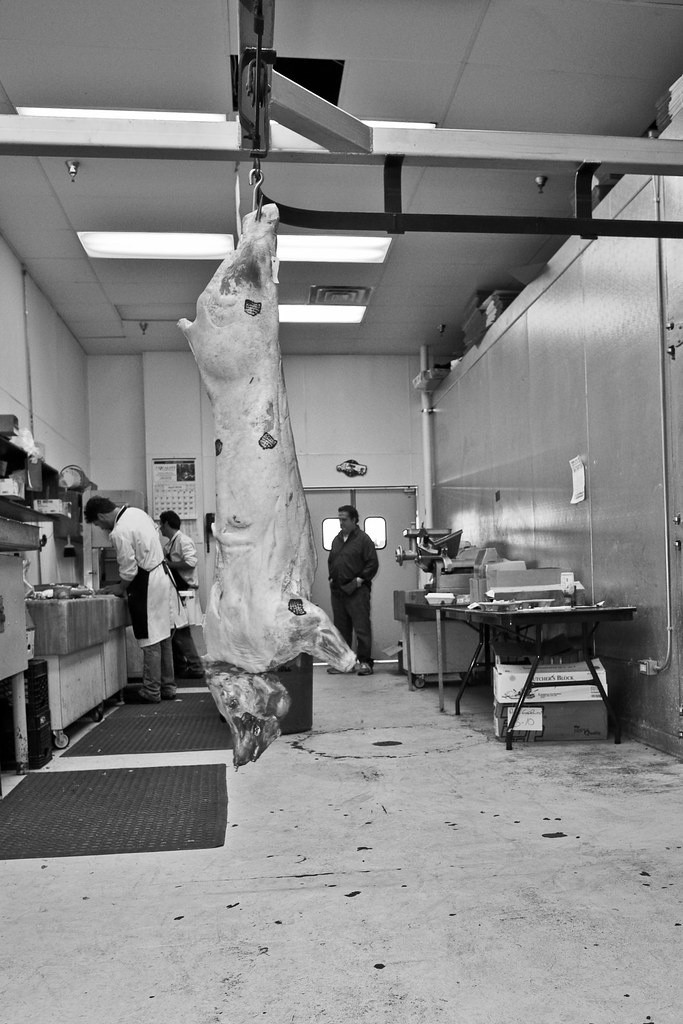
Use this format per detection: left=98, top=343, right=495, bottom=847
left=493, top=657, right=608, bottom=703
left=493, top=696, right=608, bottom=742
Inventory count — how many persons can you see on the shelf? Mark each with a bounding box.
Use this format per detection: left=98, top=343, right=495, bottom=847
left=84, top=496, right=181, bottom=702
left=157, top=511, right=206, bottom=677
left=327, top=506, right=378, bottom=676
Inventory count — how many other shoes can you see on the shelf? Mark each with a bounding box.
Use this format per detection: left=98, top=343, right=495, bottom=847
left=161, top=690, right=176, bottom=700
left=179, top=669, right=204, bottom=680
left=326, top=667, right=356, bottom=674
left=358, top=661, right=373, bottom=675
left=123, top=693, right=159, bottom=705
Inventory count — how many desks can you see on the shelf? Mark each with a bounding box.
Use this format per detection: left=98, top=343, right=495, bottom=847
left=443, top=606, right=637, bottom=749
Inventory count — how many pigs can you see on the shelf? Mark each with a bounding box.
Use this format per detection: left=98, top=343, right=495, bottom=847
left=177, top=202, right=360, bottom=770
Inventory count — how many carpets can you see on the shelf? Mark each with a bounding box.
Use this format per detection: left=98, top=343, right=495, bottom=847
left=173, top=670, right=211, bottom=688
left=0, top=763, right=228, bottom=859
left=59, top=694, right=235, bottom=758
